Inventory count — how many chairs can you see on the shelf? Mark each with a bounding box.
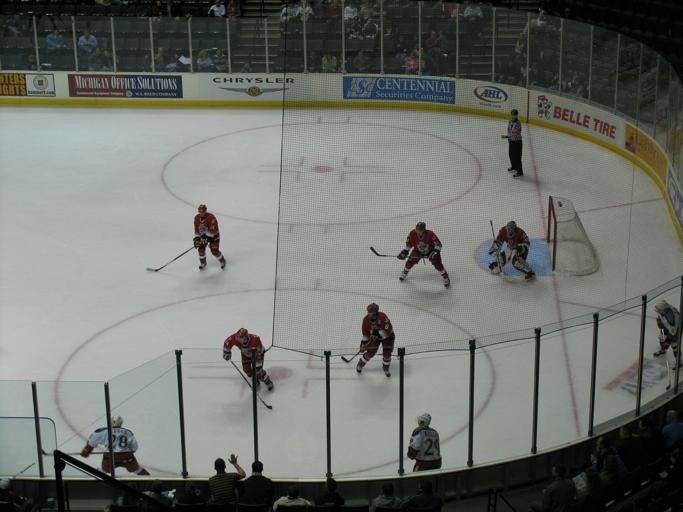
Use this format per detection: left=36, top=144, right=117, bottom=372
left=529, top=414, right=683, bottom=511
left=1, top=500, right=443, bottom=510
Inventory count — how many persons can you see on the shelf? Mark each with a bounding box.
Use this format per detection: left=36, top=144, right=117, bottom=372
left=407, top=413, right=442, bottom=472
left=653, top=300, right=682, bottom=369
left=488, top=221, right=536, bottom=281
left=116, top=459, right=344, bottom=512
left=500, top=110, right=525, bottom=177
left=398, top=221, right=450, bottom=288
left=402, top=480, right=443, bottom=512
left=531, top=410, right=683, bottom=511
left=368, top=483, right=402, bottom=511
left=356, top=302, right=395, bottom=376
left=81, top=415, right=151, bottom=476
left=193, top=203, right=227, bottom=270
left=222, top=328, right=274, bottom=392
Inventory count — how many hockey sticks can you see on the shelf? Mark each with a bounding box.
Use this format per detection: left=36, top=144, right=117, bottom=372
left=661, top=329, right=670, bottom=391
left=341, top=341, right=371, bottom=363
left=369, top=247, right=428, bottom=258
left=146, top=246, right=194, bottom=271
left=40, top=449, right=102, bottom=457
left=490, top=220, right=520, bottom=284
left=228, top=359, right=273, bottom=409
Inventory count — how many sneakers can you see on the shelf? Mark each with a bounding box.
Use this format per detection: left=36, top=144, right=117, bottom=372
left=398, top=272, right=408, bottom=280
left=653, top=349, right=666, bottom=357
left=672, top=363, right=683, bottom=371
left=507, top=167, right=523, bottom=178
left=443, top=278, right=450, bottom=287
left=250, top=380, right=274, bottom=391
left=199, top=261, right=227, bottom=269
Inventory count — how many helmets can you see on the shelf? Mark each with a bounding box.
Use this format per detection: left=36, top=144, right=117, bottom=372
left=366, top=303, right=379, bottom=313
left=111, top=415, right=123, bottom=426
left=415, top=222, right=425, bottom=231
left=654, top=299, right=669, bottom=314
left=507, top=221, right=516, bottom=229
left=197, top=204, right=206, bottom=213
left=417, top=413, right=431, bottom=427
left=235, top=328, right=248, bottom=338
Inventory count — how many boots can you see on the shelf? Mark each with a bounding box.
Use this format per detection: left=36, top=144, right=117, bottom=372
left=355, top=358, right=364, bottom=372
left=382, top=363, right=392, bottom=377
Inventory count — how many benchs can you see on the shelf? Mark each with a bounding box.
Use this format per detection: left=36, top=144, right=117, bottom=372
left=1, top=1, right=683, bottom=127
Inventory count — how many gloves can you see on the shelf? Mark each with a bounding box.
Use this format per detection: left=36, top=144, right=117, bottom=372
left=359, top=329, right=384, bottom=352
left=80, top=444, right=93, bottom=457
left=221, top=351, right=232, bottom=362
left=192, top=234, right=209, bottom=248
left=396, top=249, right=409, bottom=261
left=428, top=249, right=439, bottom=260
